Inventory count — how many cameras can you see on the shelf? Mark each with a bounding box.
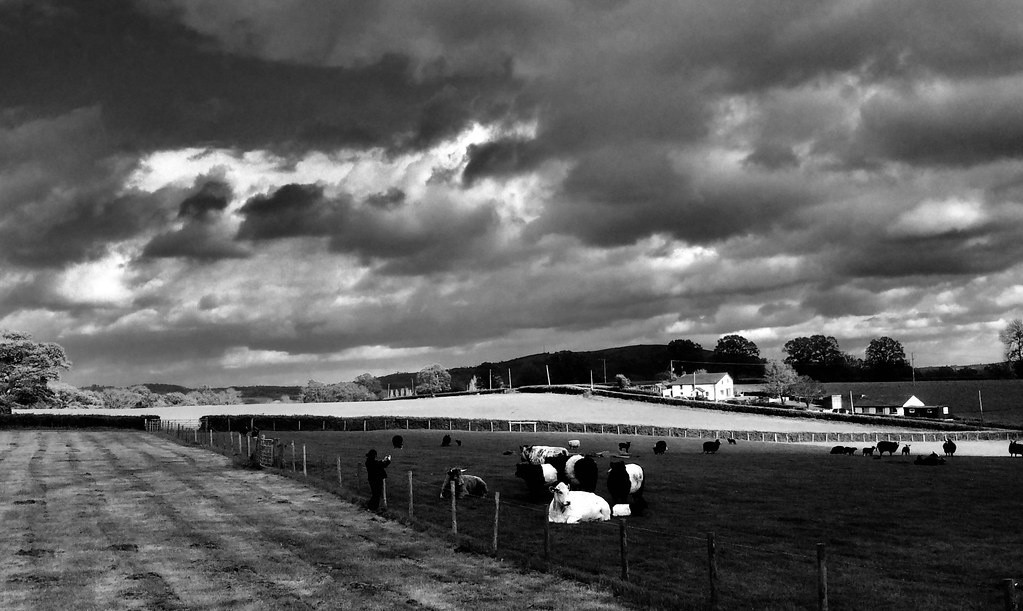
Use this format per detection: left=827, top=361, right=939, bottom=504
left=385, top=455, right=390, bottom=459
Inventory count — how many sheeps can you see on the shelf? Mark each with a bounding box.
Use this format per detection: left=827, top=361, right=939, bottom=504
left=652, top=440, right=669, bottom=455
left=514, top=439, right=645, bottom=525
left=391, top=435, right=404, bottom=448
left=439, top=465, right=490, bottom=501
left=1008, top=438, right=1023, bottom=457
left=942, top=437, right=957, bottom=457
left=441, top=433, right=463, bottom=447
left=829, top=440, right=912, bottom=462
left=702, top=438, right=722, bottom=455
left=726, top=438, right=736, bottom=445
